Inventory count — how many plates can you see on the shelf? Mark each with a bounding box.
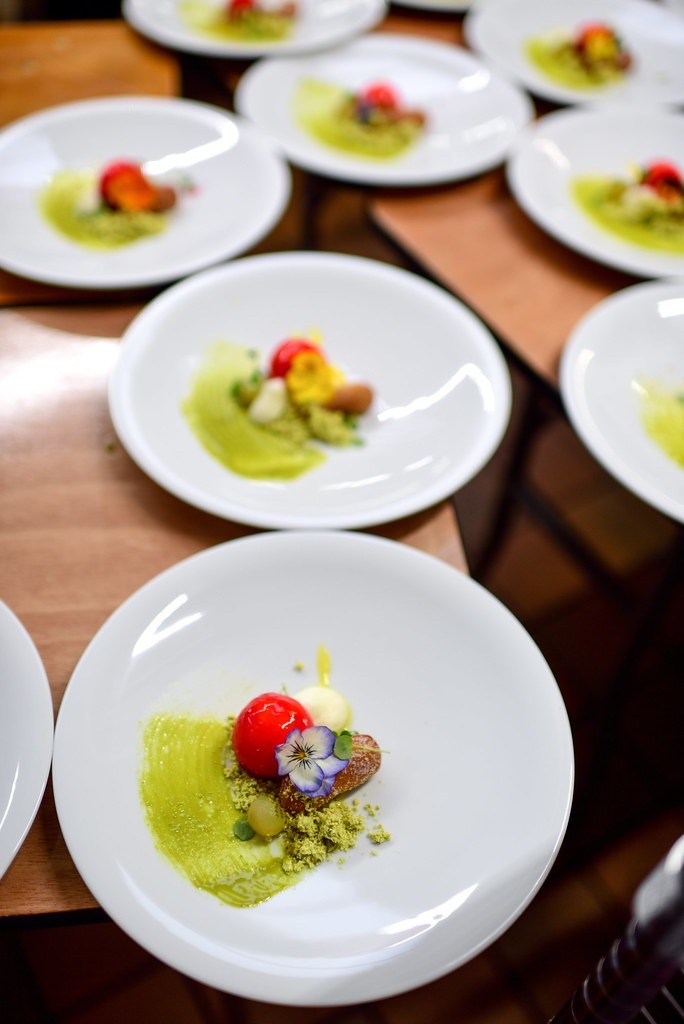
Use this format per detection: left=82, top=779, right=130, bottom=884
left=49, top=531, right=573, bottom=1007
left=127, top=0, right=385, bottom=53
left=236, top=34, right=535, bottom=188
left=0, top=99, right=289, bottom=289
left=508, top=108, right=684, bottom=277
left=108, top=250, right=511, bottom=537
left=560, top=280, right=684, bottom=526
left=0, top=602, right=55, bottom=884
left=464, top=0, right=684, bottom=107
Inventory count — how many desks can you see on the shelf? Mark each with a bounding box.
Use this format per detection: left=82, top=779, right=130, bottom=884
left=0, top=0, right=660, bottom=919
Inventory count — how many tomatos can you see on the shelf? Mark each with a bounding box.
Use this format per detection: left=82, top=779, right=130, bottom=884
left=645, top=165, right=679, bottom=190
left=368, top=88, right=394, bottom=108
left=272, top=339, right=311, bottom=375
left=232, top=692, right=313, bottom=777
left=100, top=163, right=140, bottom=210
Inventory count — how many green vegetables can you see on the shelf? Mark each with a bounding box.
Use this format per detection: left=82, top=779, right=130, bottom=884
left=332, top=730, right=391, bottom=760
